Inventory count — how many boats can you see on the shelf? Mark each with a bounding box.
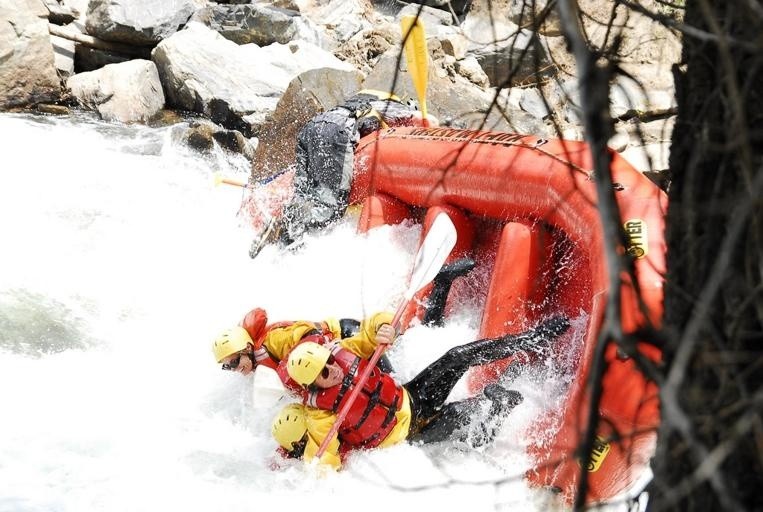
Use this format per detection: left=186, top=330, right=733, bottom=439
left=238, top=124, right=668, bottom=509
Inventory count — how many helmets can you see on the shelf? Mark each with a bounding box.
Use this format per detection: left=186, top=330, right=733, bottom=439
left=213, top=326, right=254, bottom=363
left=272, top=403, right=307, bottom=454
left=286, top=341, right=332, bottom=391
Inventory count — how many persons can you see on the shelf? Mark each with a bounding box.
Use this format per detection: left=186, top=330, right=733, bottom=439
left=210, top=258, right=570, bottom=480
left=239, top=84, right=438, bottom=259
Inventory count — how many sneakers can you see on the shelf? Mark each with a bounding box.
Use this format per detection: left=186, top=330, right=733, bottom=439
left=249, top=217, right=282, bottom=258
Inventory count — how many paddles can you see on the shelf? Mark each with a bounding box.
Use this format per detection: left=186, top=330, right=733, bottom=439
left=401, top=15, right=429, bottom=128
left=311, top=212, right=457, bottom=469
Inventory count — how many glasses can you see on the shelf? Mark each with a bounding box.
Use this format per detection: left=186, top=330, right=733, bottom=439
left=222, top=354, right=242, bottom=372
left=321, top=354, right=335, bottom=380
